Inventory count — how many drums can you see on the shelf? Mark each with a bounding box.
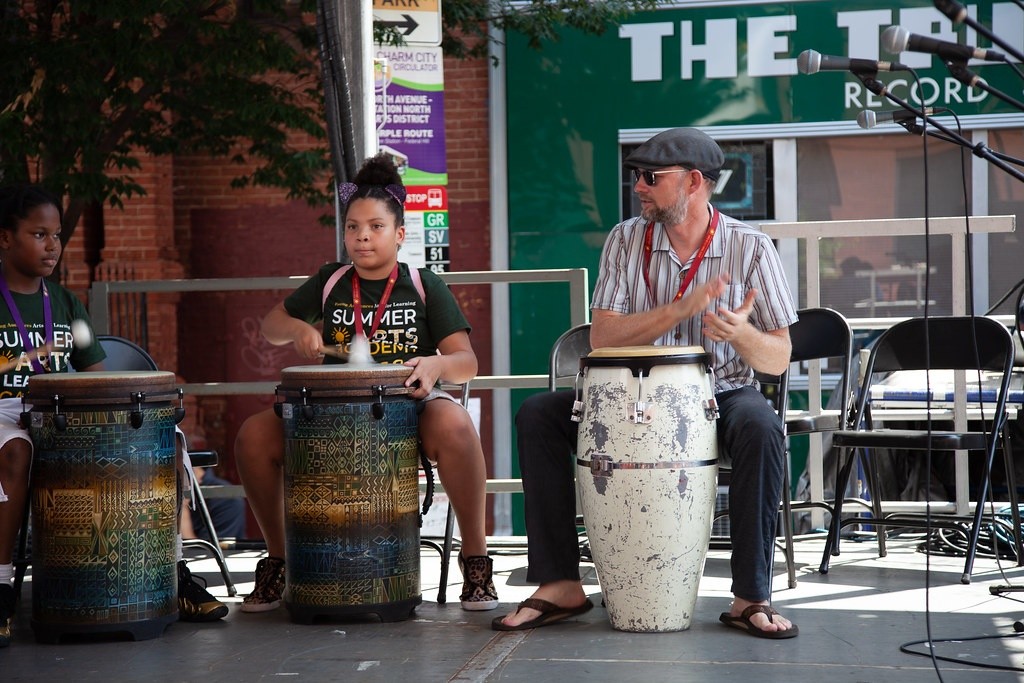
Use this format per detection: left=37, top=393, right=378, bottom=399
left=572, top=345, right=720, bottom=634
left=272, top=362, right=424, bottom=621
left=29, top=369, right=185, bottom=642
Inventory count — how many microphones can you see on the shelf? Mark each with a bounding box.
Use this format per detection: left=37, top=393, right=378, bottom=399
left=881, top=25, right=1004, bottom=62
left=857, top=108, right=948, bottom=130
left=798, top=48, right=907, bottom=75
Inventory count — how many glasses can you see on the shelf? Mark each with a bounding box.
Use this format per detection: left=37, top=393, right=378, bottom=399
left=632, top=168, right=692, bottom=186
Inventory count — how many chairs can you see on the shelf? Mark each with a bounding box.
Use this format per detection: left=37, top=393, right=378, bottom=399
left=13, top=309, right=1023, bottom=614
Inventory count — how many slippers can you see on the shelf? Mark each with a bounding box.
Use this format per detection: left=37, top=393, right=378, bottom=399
left=719, top=605, right=799, bottom=639
left=492, top=595, right=593, bottom=631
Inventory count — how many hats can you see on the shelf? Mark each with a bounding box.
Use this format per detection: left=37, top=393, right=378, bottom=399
left=624, top=127, right=725, bottom=174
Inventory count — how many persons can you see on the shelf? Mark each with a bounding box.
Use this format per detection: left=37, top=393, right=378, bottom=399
left=491, top=128, right=800, bottom=640
left=0, top=184, right=228, bottom=649
left=232, top=154, right=499, bottom=610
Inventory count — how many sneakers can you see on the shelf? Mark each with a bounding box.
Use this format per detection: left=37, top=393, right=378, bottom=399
left=177, top=560, right=229, bottom=621
left=0, top=582, right=15, bottom=642
left=241, top=556, right=286, bottom=611
left=458, top=548, right=498, bottom=610
